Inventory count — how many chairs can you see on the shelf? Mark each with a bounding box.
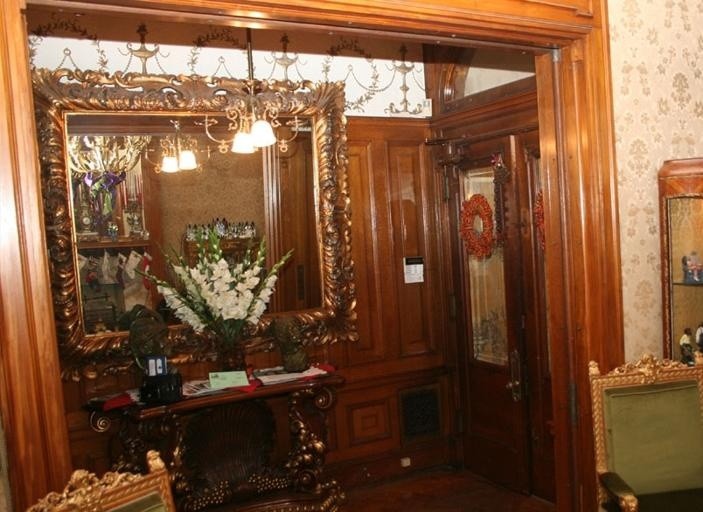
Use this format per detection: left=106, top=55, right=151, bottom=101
left=588, top=351, right=702, bottom=512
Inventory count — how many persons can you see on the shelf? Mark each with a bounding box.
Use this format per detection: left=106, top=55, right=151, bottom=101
left=678, top=248, right=702, bottom=282
left=678, top=327, right=695, bottom=368
left=694, top=320, right=703, bottom=353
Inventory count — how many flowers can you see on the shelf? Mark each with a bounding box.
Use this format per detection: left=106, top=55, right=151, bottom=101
left=175, top=226, right=295, bottom=345
left=132, top=229, right=266, bottom=324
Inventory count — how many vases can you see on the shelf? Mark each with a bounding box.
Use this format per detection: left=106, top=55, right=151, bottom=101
left=218, top=345, right=244, bottom=370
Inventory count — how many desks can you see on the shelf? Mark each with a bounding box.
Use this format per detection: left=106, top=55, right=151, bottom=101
left=79, top=359, right=347, bottom=511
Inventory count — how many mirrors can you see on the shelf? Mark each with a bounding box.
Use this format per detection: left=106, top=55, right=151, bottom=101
left=30, top=66, right=360, bottom=383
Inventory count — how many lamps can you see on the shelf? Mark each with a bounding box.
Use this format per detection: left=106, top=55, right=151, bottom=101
left=144, top=131, right=210, bottom=173
left=204, top=27, right=298, bottom=154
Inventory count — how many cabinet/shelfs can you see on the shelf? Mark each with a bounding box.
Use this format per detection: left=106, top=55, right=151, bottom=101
left=657, top=156, right=703, bottom=366
